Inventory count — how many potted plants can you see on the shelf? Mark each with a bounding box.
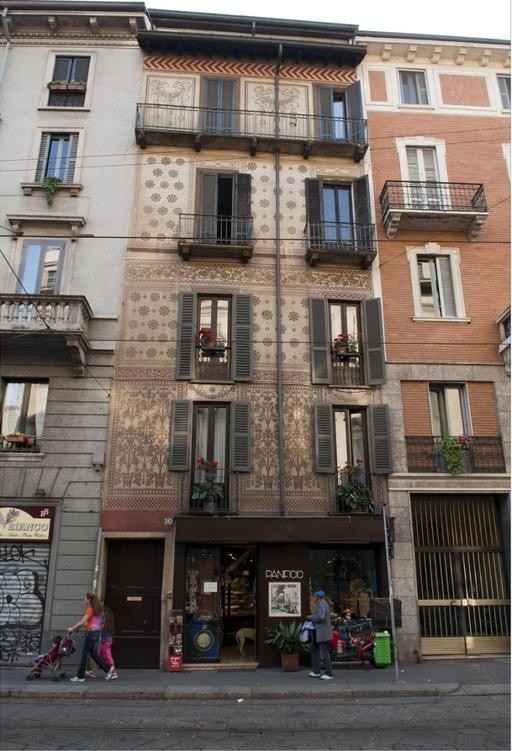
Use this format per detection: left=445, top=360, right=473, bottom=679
left=264, top=621, right=303, bottom=671
left=4, top=431, right=25, bottom=443
left=47, top=80, right=86, bottom=92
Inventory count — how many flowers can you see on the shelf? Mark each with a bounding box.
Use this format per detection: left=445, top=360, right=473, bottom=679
left=336, top=458, right=375, bottom=510
left=192, top=457, right=224, bottom=501
left=337, top=334, right=350, bottom=346
left=441, top=435, right=474, bottom=474
left=200, top=327, right=213, bottom=345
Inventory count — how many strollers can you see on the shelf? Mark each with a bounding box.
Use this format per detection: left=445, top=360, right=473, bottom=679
left=24, top=630, right=70, bottom=684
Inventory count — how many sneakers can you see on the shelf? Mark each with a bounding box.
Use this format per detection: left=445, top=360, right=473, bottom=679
left=105, top=665, right=119, bottom=680
left=70, top=676, right=85, bottom=682
left=84, top=670, right=96, bottom=678
left=309, top=672, right=334, bottom=680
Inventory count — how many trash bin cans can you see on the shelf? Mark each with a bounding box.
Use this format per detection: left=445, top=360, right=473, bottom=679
left=369, top=631, right=392, bottom=668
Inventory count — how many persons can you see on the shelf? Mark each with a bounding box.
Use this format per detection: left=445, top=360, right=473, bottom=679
left=298, top=591, right=335, bottom=681
left=67, top=592, right=114, bottom=683
left=86, top=594, right=119, bottom=679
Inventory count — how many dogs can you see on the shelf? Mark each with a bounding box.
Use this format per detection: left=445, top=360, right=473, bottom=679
left=236, top=627, right=257, bottom=657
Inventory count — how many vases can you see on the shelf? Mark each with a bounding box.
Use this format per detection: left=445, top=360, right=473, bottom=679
left=337, top=346, right=347, bottom=352
left=203, top=501, right=215, bottom=511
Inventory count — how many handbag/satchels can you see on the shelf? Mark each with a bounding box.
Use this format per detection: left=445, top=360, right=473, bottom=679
left=298, top=618, right=316, bottom=644
